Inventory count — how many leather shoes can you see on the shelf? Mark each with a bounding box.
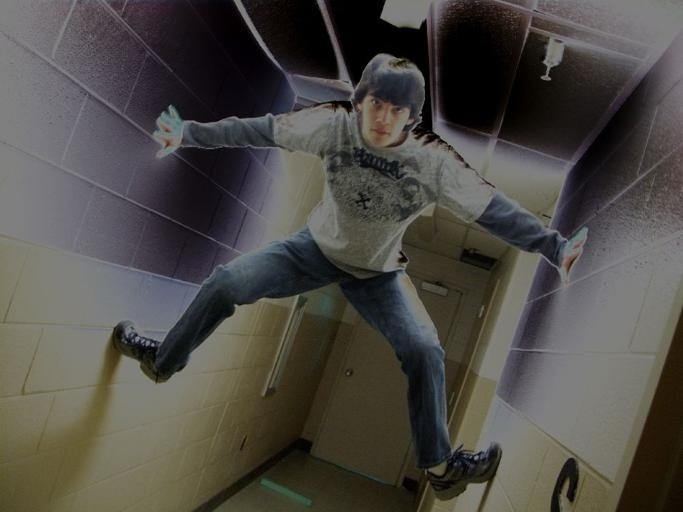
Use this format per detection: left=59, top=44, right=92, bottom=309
left=110, top=319, right=178, bottom=384
left=424, top=441, right=503, bottom=502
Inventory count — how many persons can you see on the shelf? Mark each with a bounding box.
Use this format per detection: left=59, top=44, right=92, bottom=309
left=111, top=53, right=587, bottom=502
